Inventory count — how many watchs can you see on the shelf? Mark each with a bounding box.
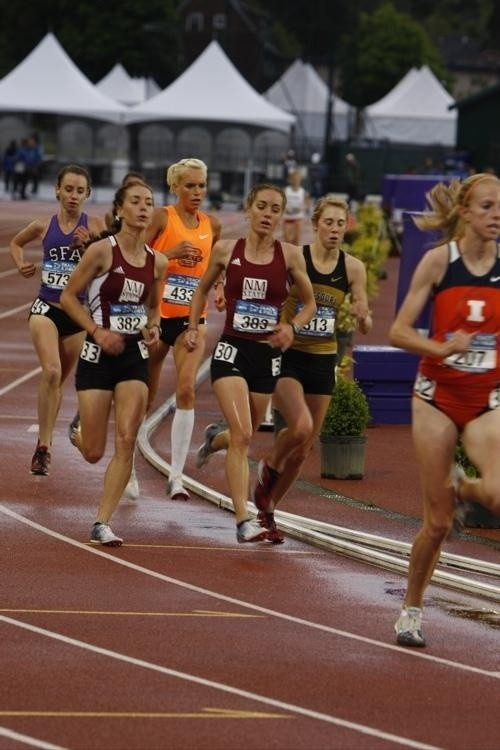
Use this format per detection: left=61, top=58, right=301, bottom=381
left=286, top=318, right=303, bottom=336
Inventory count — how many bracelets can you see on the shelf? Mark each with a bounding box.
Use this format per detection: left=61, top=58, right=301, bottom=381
left=91, top=325, right=99, bottom=337
left=213, top=280, right=224, bottom=291
left=183, top=326, right=202, bottom=335
left=150, top=323, right=163, bottom=338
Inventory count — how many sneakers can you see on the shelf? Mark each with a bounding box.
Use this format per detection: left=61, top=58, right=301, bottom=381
left=194, top=421, right=224, bottom=468
left=123, top=461, right=141, bottom=500
left=257, top=509, right=285, bottom=545
left=165, top=477, right=191, bottom=500
left=236, top=515, right=270, bottom=543
left=89, top=520, right=127, bottom=549
left=253, top=454, right=283, bottom=512
left=32, top=438, right=53, bottom=476
left=443, top=462, right=470, bottom=540
left=392, top=603, right=426, bottom=647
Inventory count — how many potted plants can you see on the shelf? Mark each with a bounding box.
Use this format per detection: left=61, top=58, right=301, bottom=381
left=455, top=429, right=500, bottom=529
left=320, top=368, right=367, bottom=479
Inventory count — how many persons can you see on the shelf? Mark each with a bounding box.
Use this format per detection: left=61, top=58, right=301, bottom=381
left=385, top=170, right=500, bottom=646
left=8, top=161, right=111, bottom=477
left=125, top=156, right=228, bottom=503
left=179, top=184, right=318, bottom=545
left=255, top=198, right=372, bottom=547
left=0, top=127, right=46, bottom=200
left=104, top=170, right=144, bottom=228
left=59, top=183, right=169, bottom=550
left=275, top=147, right=500, bottom=198
left=281, top=169, right=312, bottom=245
